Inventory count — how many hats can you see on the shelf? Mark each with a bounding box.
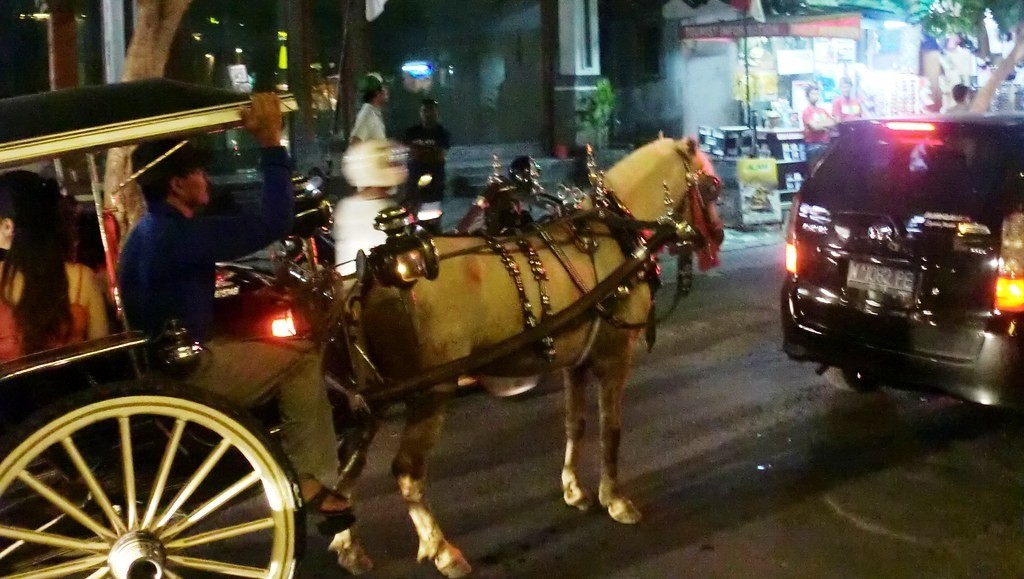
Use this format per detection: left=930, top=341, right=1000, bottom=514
left=129, top=139, right=216, bottom=187
left=356, top=71, right=394, bottom=91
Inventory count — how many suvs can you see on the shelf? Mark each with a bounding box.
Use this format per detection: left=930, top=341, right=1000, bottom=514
left=779, top=112, right=1023, bottom=418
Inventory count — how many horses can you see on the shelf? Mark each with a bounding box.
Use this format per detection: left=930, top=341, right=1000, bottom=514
left=316, top=126, right=726, bottom=579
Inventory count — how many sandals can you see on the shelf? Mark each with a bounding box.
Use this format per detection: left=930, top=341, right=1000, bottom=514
left=304, top=486, right=351, bottom=517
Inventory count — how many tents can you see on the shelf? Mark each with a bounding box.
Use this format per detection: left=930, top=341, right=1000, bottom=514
left=680, top=0, right=859, bottom=127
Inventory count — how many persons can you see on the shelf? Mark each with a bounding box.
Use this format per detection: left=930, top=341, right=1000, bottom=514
left=402, top=99, right=453, bottom=238
left=947, top=84, right=968, bottom=114
left=0, top=170, right=107, bottom=366
left=117, top=90, right=354, bottom=515
left=801, top=74, right=861, bottom=173
left=348, top=74, right=389, bottom=145
left=334, top=139, right=410, bottom=286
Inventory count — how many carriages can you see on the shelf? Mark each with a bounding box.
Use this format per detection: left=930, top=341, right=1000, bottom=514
left=0, top=78, right=726, bottom=579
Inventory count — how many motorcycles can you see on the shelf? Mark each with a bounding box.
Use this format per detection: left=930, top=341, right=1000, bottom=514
left=321, top=172, right=550, bottom=400
